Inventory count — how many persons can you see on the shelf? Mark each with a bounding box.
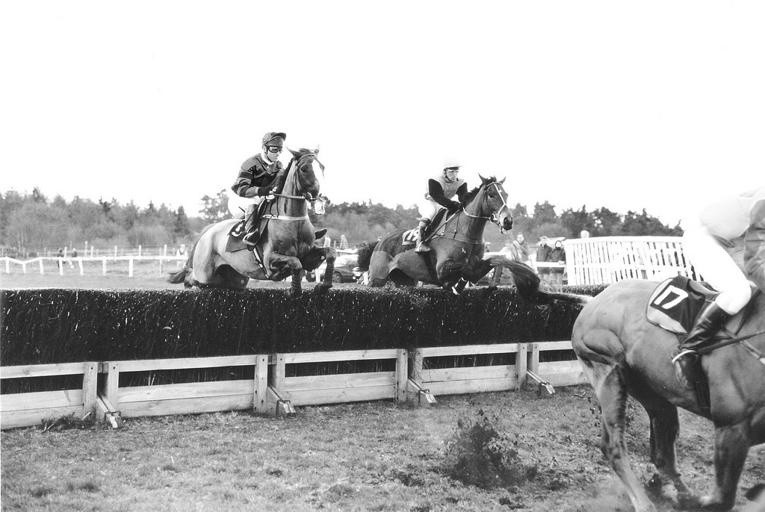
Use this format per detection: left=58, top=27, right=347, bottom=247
left=176, top=244, right=188, bottom=268
left=57, top=248, right=77, bottom=268
left=670, top=189, right=765, bottom=390
left=511, top=233, right=566, bottom=284
left=228, top=131, right=327, bottom=251
left=415, top=167, right=467, bottom=252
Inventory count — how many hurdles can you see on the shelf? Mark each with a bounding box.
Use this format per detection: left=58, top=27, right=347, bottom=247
left=0, top=284, right=609, bottom=429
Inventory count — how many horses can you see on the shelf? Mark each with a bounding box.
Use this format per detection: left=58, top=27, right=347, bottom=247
left=497, top=260, right=765, bottom=512
left=357, top=173, right=513, bottom=302
left=168, top=147, right=335, bottom=300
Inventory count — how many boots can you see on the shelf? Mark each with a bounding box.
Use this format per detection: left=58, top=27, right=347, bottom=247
left=315, top=226, right=326, bottom=241
left=244, top=204, right=260, bottom=247
left=415, top=226, right=433, bottom=253
left=672, top=299, right=737, bottom=391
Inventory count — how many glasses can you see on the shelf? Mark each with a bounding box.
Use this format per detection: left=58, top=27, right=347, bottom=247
left=268, top=146, right=283, bottom=153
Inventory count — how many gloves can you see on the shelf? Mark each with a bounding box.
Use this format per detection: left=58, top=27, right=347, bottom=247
left=258, top=185, right=277, bottom=196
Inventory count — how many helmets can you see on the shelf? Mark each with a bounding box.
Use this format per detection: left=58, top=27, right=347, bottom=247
left=442, top=158, right=460, bottom=171
left=262, top=131, right=286, bottom=143
